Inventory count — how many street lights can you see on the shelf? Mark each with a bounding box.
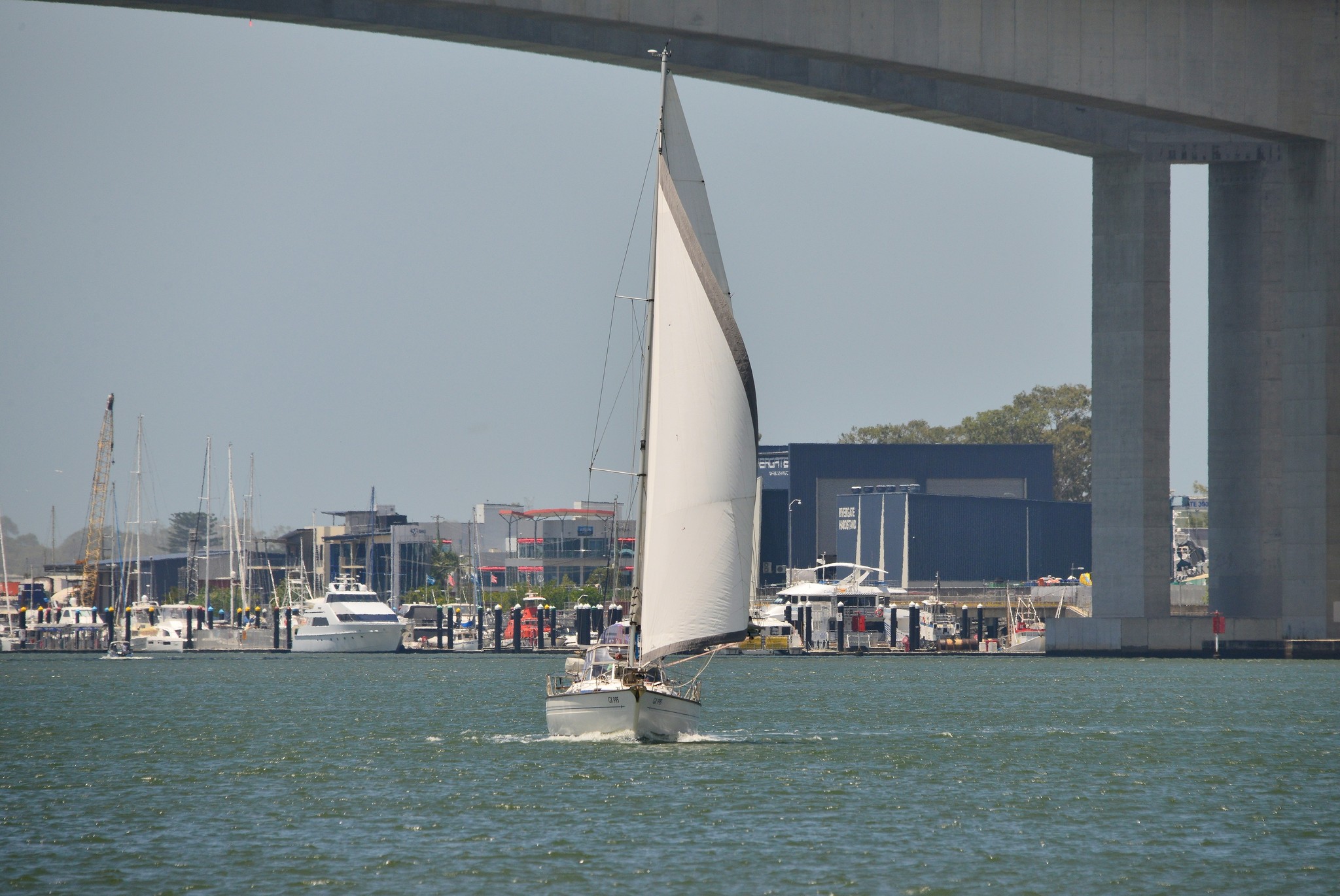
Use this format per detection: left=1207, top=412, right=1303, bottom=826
left=789, top=499, right=802, bottom=586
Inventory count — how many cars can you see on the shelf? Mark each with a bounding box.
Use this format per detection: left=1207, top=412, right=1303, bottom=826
left=0, top=605, right=18, bottom=615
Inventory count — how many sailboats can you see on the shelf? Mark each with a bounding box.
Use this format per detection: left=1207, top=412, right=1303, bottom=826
left=118, top=413, right=255, bottom=653
left=545, top=40, right=760, bottom=745
left=397, top=507, right=571, bottom=650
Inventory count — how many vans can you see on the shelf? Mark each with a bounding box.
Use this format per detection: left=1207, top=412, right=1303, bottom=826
left=55, top=607, right=108, bottom=639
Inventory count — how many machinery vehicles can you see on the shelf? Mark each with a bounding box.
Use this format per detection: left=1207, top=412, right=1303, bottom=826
left=76, top=393, right=115, bottom=607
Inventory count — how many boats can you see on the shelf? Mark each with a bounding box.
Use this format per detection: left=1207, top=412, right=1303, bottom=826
left=106, top=641, right=133, bottom=659
left=920, top=570, right=959, bottom=643
left=708, top=578, right=806, bottom=656
left=266, top=485, right=407, bottom=653
left=1002, top=578, right=1046, bottom=653
